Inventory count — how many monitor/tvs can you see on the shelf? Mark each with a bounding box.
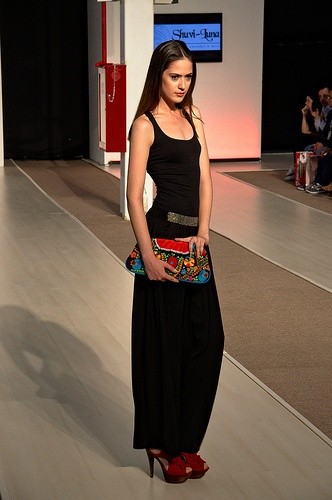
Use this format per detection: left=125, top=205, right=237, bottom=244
left=153, top=13, right=223, bottom=63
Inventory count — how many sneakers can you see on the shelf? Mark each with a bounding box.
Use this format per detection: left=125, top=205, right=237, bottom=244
left=298, top=185, right=305, bottom=190
left=306, top=185, right=321, bottom=193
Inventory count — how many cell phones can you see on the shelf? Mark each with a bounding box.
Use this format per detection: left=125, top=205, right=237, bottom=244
left=312, top=102, right=316, bottom=111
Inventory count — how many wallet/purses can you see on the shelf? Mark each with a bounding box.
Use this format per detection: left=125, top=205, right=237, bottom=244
left=125, top=238, right=211, bottom=283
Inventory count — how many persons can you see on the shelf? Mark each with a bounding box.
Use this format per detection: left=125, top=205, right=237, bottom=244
left=283, top=86, right=332, bottom=194
left=127, top=39, right=224, bottom=484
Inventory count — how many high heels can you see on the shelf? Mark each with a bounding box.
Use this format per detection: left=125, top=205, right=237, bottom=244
left=146, top=449, right=209, bottom=483
